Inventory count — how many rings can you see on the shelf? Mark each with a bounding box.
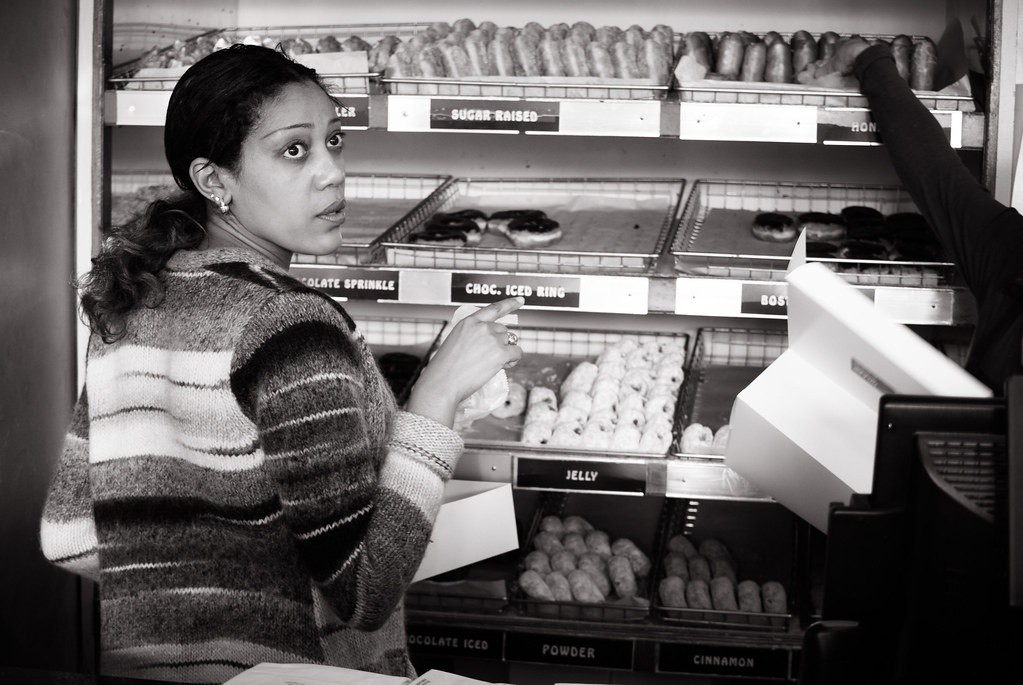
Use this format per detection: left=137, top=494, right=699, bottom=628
left=507, top=330, right=518, bottom=346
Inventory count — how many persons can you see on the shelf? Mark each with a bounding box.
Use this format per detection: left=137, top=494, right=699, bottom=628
left=40, top=45, right=526, bottom=685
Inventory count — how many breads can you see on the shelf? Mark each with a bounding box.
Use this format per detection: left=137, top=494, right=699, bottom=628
left=134, top=17, right=940, bottom=97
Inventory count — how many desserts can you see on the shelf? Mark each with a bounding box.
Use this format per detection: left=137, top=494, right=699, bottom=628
left=408, top=206, right=941, bottom=269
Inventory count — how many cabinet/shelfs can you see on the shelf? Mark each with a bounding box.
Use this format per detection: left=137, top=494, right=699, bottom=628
left=103, top=2, right=1023, bottom=681
